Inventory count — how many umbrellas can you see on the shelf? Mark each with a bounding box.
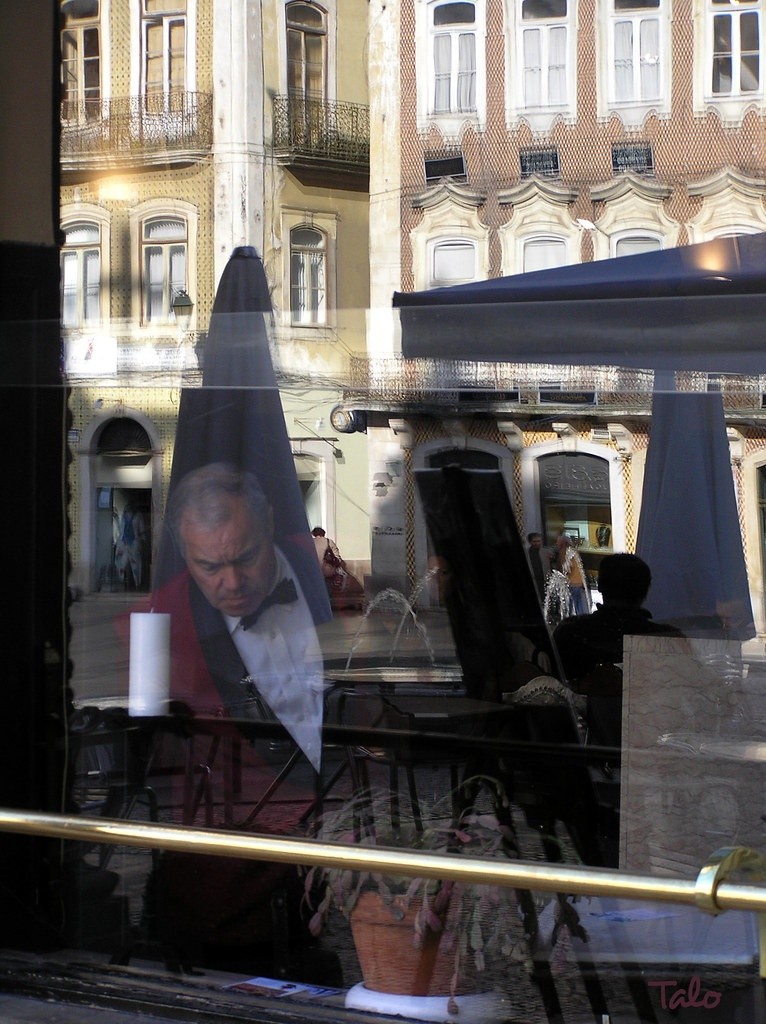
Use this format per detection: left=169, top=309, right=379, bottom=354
left=630, top=368, right=754, bottom=642
left=155, top=248, right=332, bottom=619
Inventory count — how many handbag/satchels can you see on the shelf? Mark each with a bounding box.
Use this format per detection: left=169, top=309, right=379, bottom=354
left=324, top=538, right=339, bottom=567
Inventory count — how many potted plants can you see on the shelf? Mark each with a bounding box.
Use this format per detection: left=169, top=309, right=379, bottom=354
left=295, top=774, right=535, bottom=1016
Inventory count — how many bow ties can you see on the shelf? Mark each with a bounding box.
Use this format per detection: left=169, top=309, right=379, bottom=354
left=237, top=577, right=299, bottom=632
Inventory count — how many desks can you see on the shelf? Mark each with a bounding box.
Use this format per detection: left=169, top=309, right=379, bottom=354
left=63, top=668, right=469, bottom=847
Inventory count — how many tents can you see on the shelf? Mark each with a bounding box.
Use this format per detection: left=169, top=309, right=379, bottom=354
left=391, top=231, right=766, bottom=394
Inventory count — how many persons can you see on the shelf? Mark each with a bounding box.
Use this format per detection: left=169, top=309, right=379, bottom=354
left=595, top=523, right=611, bottom=548
left=113, top=502, right=147, bottom=591
left=118, top=462, right=358, bottom=989
left=557, top=536, right=591, bottom=616
left=550, top=555, right=693, bottom=849
left=525, top=530, right=555, bottom=610
left=312, top=526, right=348, bottom=619
